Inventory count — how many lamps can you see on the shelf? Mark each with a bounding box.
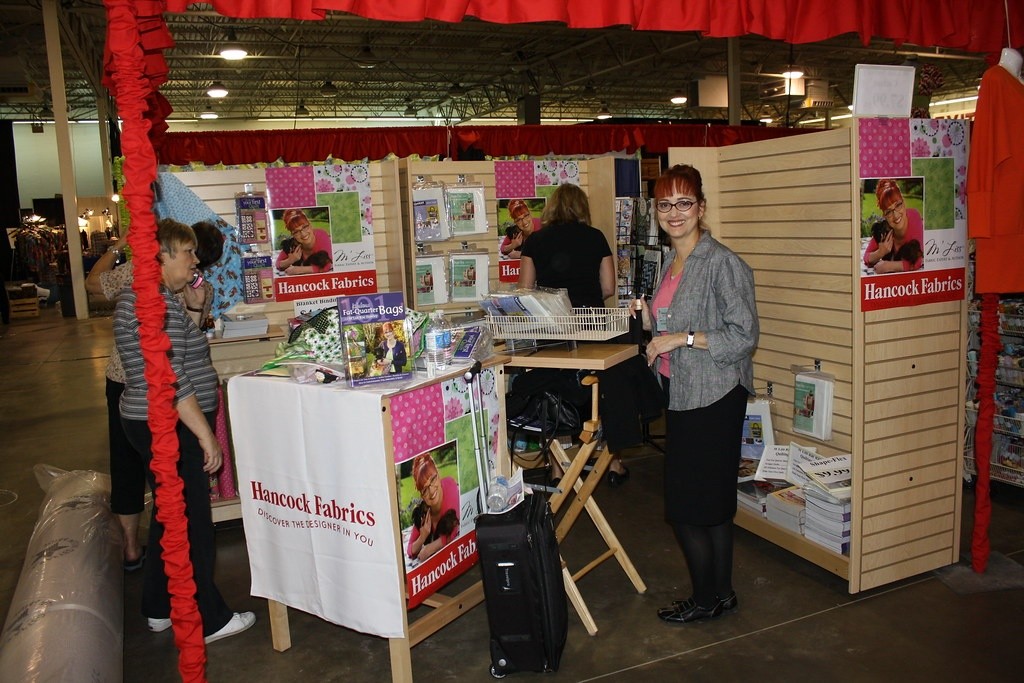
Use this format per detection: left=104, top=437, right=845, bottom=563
left=598, top=105, right=612, bottom=117
left=39, top=108, right=54, bottom=117
left=201, top=106, right=218, bottom=118
left=669, top=86, right=688, bottom=103
left=404, top=107, right=417, bottom=115
left=356, top=47, right=377, bottom=68
left=207, top=81, right=228, bottom=97
left=320, top=83, right=338, bottom=97
left=448, top=84, right=465, bottom=96
left=31, top=122, right=45, bottom=134
left=220, top=31, right=247, bottom=60
left=295, top=106, right=309, bottom=116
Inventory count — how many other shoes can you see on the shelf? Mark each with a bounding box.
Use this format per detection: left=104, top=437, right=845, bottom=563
left=124, top=545, right=148, bottom=570
left=608, top=463, right=629, bottom=486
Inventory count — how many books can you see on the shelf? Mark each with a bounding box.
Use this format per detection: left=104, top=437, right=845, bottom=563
left=737, top=371, right=850, bottom=556
left=337, top=291, right=412, bottom=388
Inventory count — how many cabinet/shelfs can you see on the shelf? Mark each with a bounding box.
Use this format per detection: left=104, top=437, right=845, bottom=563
left=963, top=258, right=1024, bottom=492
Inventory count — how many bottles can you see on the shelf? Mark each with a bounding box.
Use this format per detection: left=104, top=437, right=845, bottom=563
left=436, top=309, right=451, bottom=365
left=426, top=313, right=446, bottom=372
left=487, top=474, right=509, bottom=510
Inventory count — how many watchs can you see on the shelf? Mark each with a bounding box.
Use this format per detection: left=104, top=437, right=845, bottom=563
left=687, top=331, right=695, bottom=347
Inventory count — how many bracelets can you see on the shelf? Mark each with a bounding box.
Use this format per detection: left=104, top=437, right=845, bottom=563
left=187, top=305, right=203, bottom=313
left=108, top=246, right=121, bottom=263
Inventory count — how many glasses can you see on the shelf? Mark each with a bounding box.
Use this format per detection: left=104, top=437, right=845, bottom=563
left=384, top=330, right=392, bottom=334
left=421, top=473, right=438, bottom=497
left=514, top=211, right=530, bottom=224
left=656, top=197, right=703, bottom=213
left=291, top=221, right=310, bottom=236
left=882, top=199, right=903, bottom=217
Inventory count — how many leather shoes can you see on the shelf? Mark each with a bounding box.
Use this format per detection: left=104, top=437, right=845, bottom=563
left=657, top=595, right=721, bottom=623
left=672, top=590, right=737, bottom=610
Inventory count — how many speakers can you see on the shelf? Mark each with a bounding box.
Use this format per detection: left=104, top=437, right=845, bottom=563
left=516, top=95, right=540, bottom=125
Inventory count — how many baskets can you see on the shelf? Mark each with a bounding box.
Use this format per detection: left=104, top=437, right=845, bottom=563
left=485, top=308, right=631, bottom=342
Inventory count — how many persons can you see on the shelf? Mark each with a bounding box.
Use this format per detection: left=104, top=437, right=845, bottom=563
left=863, top=179, right=924, bottom=274
left=517, top=183, right=615, bottom=344
left=407, top=453, right=460, bottom=562
left=630, top=164, right=759, bottom=622
left=114, top=219, right=256, bottom=645
left=502, top=199, right=541, bottom=259
left=85, top=221, right=226, bottom=569
left=968, top=49, right=1024, bottom=293
left=375, top=323, right=407, bottom=374
left=276, top=207, right=333, bottom=276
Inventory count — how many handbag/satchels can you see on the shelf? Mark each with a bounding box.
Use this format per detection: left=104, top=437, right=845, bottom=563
left=508, top=389, right=584, bottom=461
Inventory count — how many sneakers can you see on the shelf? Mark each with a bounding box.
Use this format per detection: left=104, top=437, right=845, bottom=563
left=148, top=617, right=172, bottom=632
left=204, top=612, right=256, bottom=644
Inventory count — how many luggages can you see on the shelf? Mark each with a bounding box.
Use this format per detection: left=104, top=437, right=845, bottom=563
left=464, top=361, right=570, bottom=679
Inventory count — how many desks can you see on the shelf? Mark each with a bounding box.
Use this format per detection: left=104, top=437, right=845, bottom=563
left=225, top=356, right=513, bottom=683
left=493, top=339, right=646, bottom=635
